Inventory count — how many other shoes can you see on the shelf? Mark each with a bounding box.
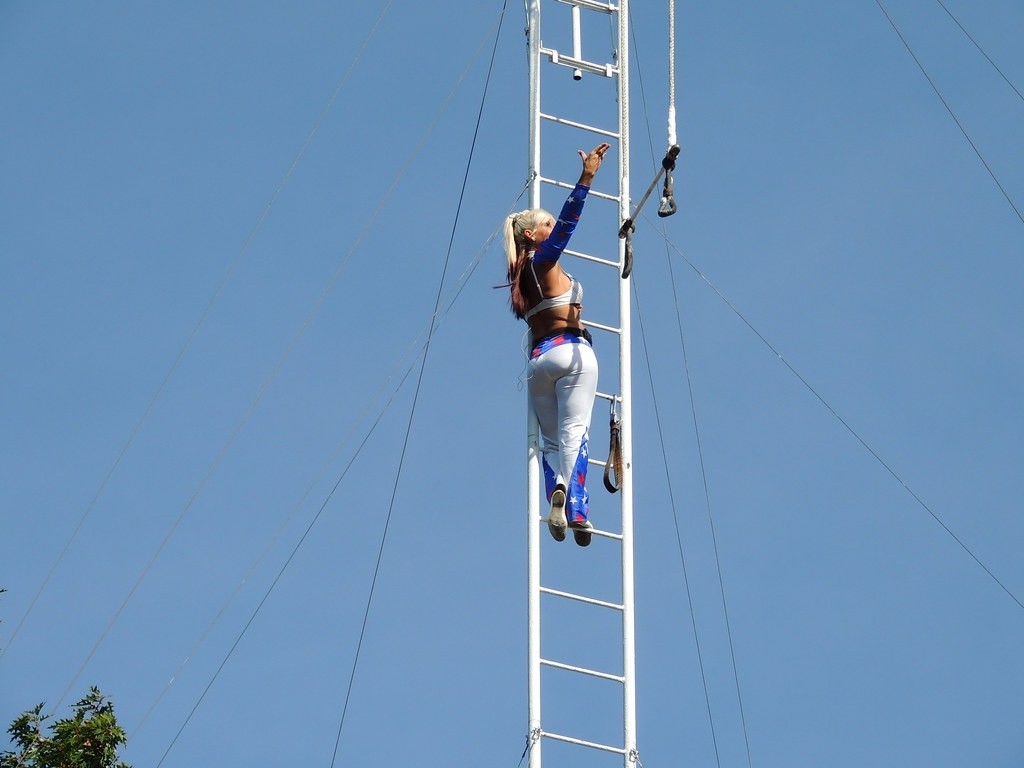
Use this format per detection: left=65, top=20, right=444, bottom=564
left=547, top=490, right=568, bottom=543
left=570, top=518, right=593, bottom=548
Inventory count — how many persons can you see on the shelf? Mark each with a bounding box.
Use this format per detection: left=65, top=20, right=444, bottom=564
left=504, top=143, right=611, bottom=547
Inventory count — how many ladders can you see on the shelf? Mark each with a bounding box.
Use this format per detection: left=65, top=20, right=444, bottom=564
left=528, top=0, right=638, bottom=768
